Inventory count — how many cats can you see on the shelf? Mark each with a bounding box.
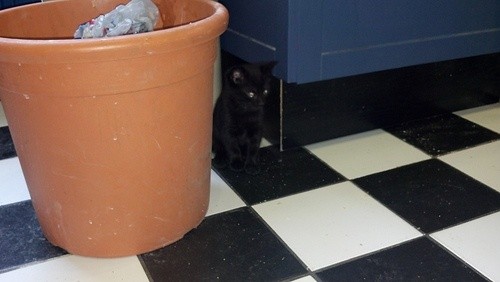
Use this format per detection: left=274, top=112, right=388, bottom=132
left=212, top=60, right=279, bottom=171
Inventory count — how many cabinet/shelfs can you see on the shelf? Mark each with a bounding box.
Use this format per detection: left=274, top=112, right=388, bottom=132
left=216, top=0, right=500, bottom=151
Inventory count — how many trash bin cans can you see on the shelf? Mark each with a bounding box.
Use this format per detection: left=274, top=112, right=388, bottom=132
left=0, top=0, right=230, bottom=260
